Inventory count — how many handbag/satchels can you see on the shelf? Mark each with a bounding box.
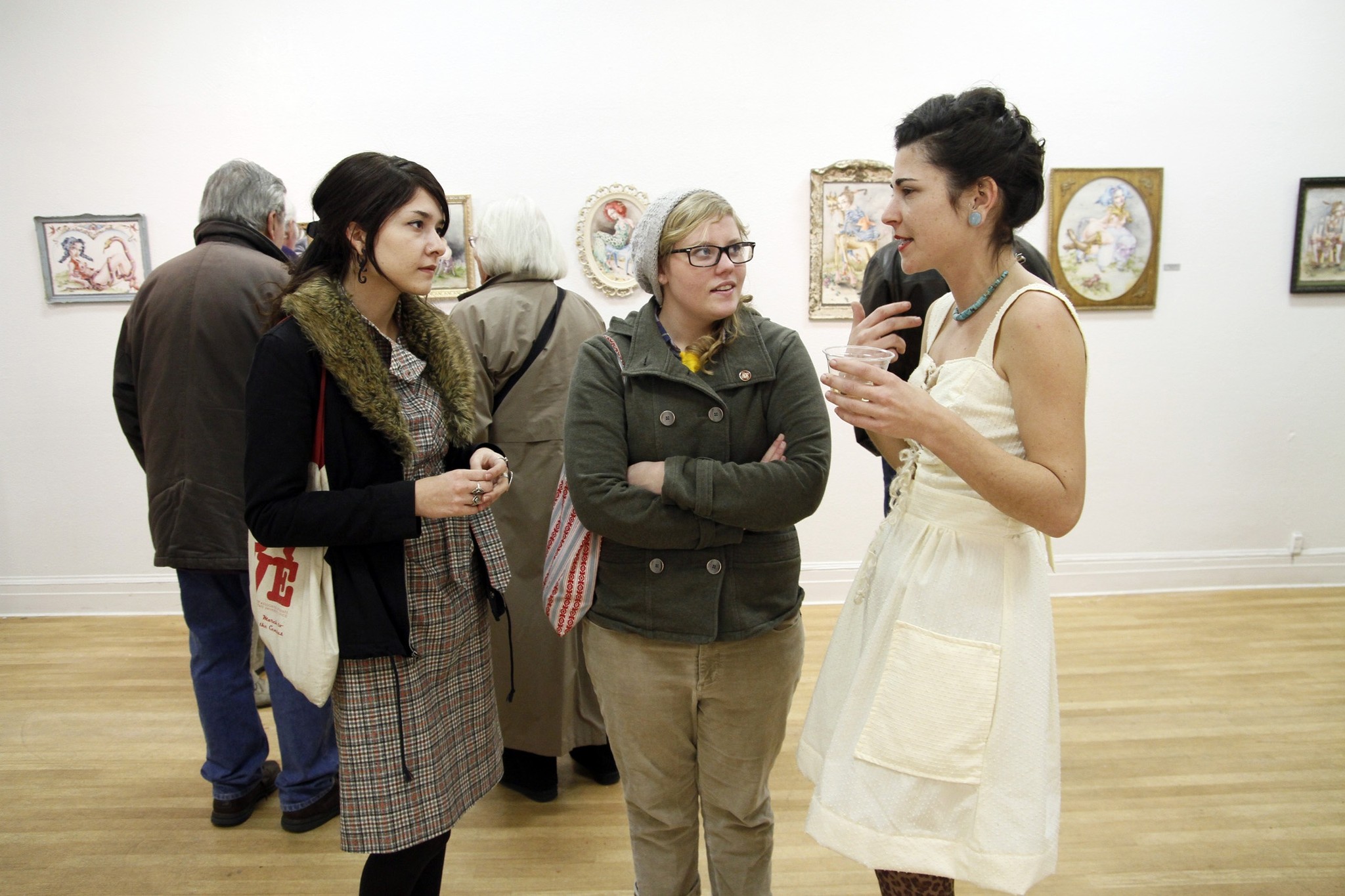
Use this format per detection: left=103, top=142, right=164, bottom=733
left=248, top=464, right=340, bottom=707
left=543, top=462, right=601, bottom=636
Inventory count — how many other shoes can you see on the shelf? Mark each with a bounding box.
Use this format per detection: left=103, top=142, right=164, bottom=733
left=570, top=751, right=620, bottom=784
left=211, top=760, right=281, bottom=826
left=498, top=766, right=557, bottom=802
left=281, top=782, right=340, bottom=833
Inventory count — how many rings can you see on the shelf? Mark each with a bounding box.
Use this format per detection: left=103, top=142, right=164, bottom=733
left=497, top=455, right=509, bottom=464
left=469, top=493, right=480, bottom=507
left=469, top=481, right=485, bottom=495
left=497, top=469, right=514, bottom=493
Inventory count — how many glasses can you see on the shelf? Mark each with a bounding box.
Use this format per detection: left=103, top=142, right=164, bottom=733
left=468, top=236, right=479, bottom=248
left=665, top=242, right=755, bottom=267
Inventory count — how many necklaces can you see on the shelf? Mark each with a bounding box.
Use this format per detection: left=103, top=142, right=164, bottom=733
left=952, top=252, right=1023, bottom=322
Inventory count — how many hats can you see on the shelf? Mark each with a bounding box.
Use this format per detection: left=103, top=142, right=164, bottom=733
left=630, top=188, right=725, bottom=307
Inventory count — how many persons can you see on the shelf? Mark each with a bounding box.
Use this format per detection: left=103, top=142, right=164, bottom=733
left=242, top=151, right=514, bottom=896
left=851, top=229, right=1060, bottom=521
left=109, top=159, right=347, bottom=838
left=795, top=83, right=1091, bottom=896
left=449, top=197, right=622, bottom=800
left=561, top=181, right=835, bottom=894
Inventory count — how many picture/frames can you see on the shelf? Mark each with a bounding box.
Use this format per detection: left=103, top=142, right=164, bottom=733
left=416, top=193, right=478, bottom=300
left=1050, top=166, right=1163, bottom=310
left=809, top=159, right=896, bottom=323
left=32, top=214, right=156, bottom=307
left=292, top=220, right=315, bottom=258
left=576, top=184, right=651, bottom=300
left=1290, top=176, right=1345, bottom=293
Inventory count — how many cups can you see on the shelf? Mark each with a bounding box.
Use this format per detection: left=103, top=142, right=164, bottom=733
left=823, top=345, right=895, bottom=416
